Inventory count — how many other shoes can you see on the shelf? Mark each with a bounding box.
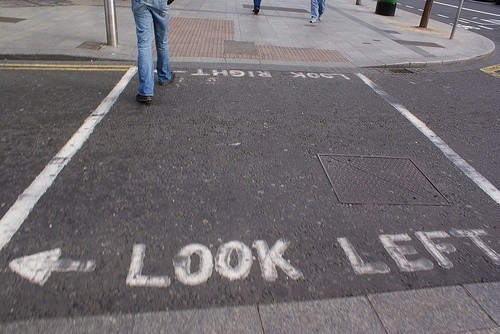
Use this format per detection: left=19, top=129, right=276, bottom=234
left=159, top=72, right=176, bottom=85
left=318, top=14, right=323, bottom=20
left=253, top=7, right=259, bottom=15
left=310, top=19, right=316, bottom=23
left=136, top=94, right=153, bottom=101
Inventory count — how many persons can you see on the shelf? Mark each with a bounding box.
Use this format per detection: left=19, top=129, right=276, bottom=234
left=252, top=0, right=262, bottom=15
left=132, top=0, right=175, bottom=104
left=309, top=0, right=325, bottom=23
